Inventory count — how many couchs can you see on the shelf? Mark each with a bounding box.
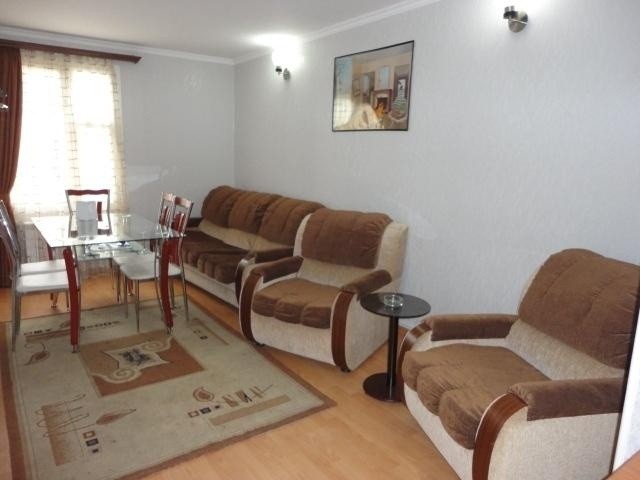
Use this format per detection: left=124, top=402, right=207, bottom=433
left=397, top=249, right=639, bottom=479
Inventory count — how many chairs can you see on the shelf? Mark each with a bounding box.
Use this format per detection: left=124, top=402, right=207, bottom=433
left=0, top=188, right=195, bottom=354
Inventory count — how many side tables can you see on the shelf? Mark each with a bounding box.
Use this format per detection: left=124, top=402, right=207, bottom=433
left=360, top=292, right=431, bottom=402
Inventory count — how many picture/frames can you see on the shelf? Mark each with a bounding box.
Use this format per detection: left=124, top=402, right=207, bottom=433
left=332, top=40, right=414, bottom=131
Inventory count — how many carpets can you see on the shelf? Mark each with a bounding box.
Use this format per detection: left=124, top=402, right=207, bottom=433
left=1, top=291, right=337, bottom=480
